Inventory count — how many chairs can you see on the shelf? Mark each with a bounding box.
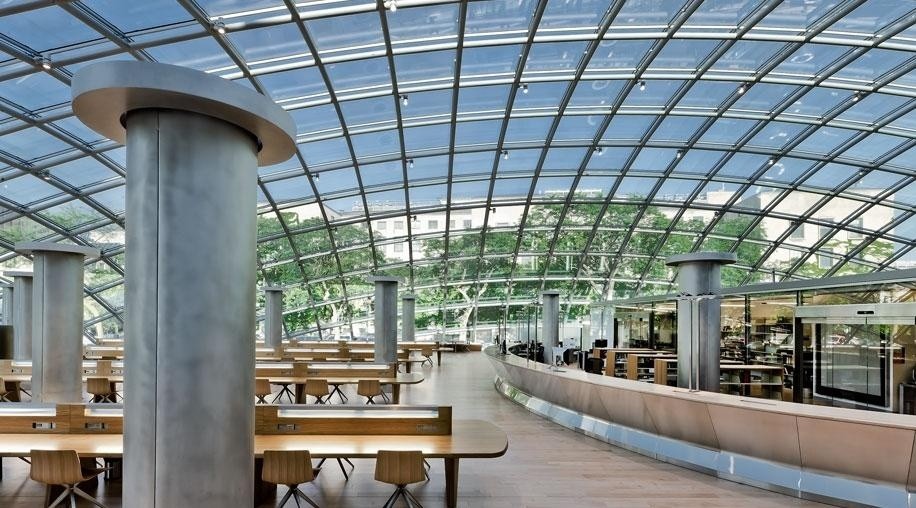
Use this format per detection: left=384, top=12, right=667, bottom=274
left=588, top=340, right=608, bottom=354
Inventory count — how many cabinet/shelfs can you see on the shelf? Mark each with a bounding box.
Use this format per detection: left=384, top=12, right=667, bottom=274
left=899, top=383, right=915, bottom=415
left=594, top=348, right=785, bottom=401
